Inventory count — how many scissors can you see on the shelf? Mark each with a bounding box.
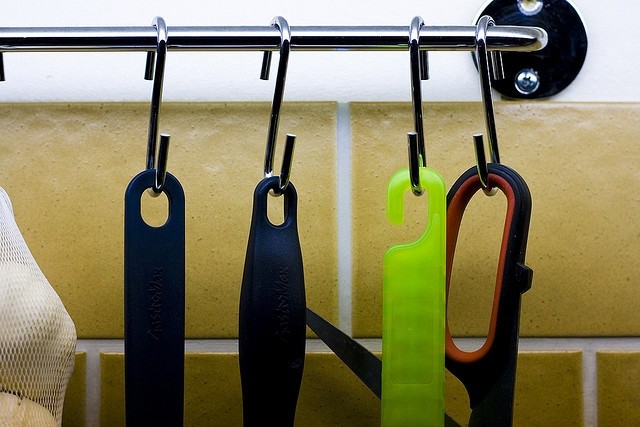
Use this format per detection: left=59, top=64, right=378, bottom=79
left=307, top=164, right=533, bottom=426
